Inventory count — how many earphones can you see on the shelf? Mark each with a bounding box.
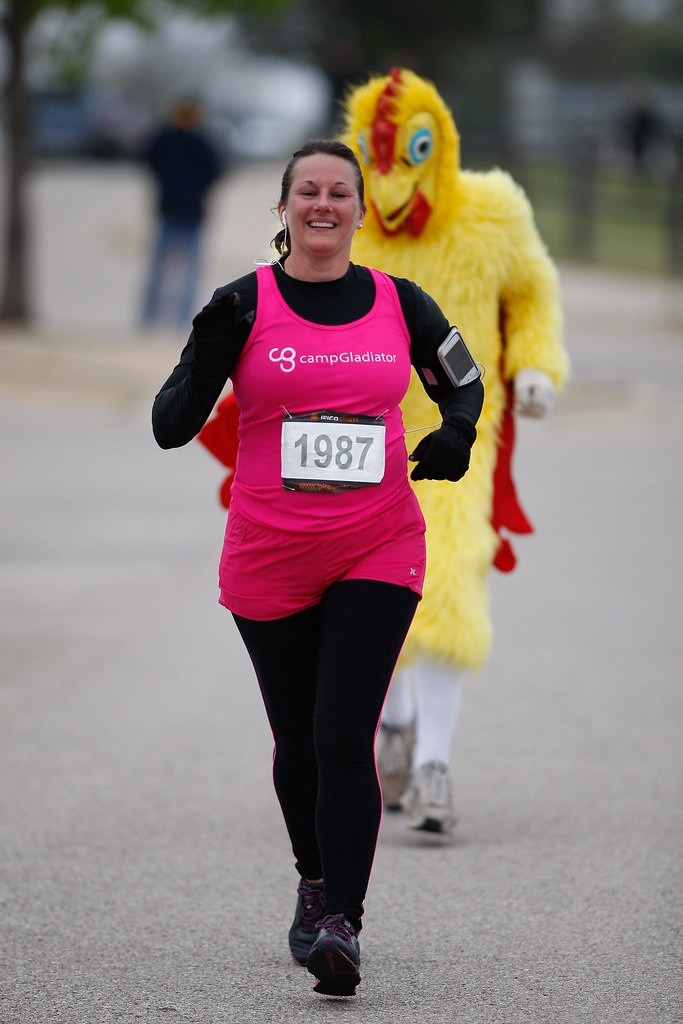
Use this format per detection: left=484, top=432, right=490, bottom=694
left=282, top=211, right=286, bottom=226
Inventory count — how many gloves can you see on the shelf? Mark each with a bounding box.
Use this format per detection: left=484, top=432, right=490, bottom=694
left=193, top=294, right=239, bottom=366
left=514, top=370, right=554, bottom=418
left=409, top=417, right=476, bottom=483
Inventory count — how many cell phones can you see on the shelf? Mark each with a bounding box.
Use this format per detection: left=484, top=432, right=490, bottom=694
left=436, top=325, right=481, bottom=388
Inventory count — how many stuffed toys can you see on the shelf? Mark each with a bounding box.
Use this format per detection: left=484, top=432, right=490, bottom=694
left=334, top=64, right=579, bottom=833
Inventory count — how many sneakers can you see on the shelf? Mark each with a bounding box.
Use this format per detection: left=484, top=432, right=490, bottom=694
left=289, top=879, right=323, bottom=965
left=410, top=760, right=458, bottom=832
left=309, top=915, right=360, bottom=996
left=377, top=723, right=413, bottom=807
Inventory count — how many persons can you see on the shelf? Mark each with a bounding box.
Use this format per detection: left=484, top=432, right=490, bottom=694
left=124, top=82, right=234, bottom=341
left=146, top=138, right=491, bottom=1003
